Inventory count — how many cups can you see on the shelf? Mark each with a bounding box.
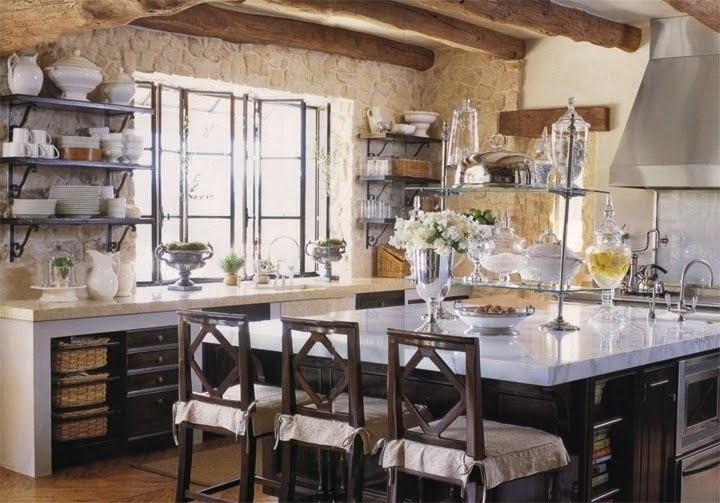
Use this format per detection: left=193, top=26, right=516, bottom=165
left=30, top=129, right=52, bottom=144
left=39, top=144, right=59, bottom=157
left=420, top=249, right=460, bottom=319
left=2, top=141, right=29, bottom=156
left=13, top=127, right=33, bottom=143
left=360, top=199, right=391, bottom=218
left=25, top=143, right=47, bottom=157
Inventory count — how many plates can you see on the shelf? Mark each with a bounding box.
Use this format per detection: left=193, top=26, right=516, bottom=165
left=51, top=134, right=100, bottom=148
left=11, top=198, right=59, bottom=217
left=29, top=283, right=88, bottom=302
left=48, top=185, right=102, bottom=218
left=454, top=300, right=535, bottom=335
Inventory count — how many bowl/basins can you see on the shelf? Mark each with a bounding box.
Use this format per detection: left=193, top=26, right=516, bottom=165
left=88, top=126, right=143, bottom=163
left=392, top=123, right=417, bottom=135
left=99, top=185, right=127, bottom=218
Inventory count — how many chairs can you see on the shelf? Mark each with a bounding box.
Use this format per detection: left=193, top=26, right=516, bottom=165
left=169, top=309, right=316, bottom=498
left=383, top=326, right=566, bottom=498
left=275, top=314, right=427, bottom=498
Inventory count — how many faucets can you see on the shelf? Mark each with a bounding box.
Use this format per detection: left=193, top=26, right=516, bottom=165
left=255, top=235, right=299, bottom=285
left=665, top=259, right=714, bottom=322
left=649, top=279, right=664, bottom=320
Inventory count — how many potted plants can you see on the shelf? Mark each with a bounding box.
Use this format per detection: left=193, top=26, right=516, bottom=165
left=157, top=146, right=214, bottom=292
left=220, top=248, right=245, bottom=286
left=307, top=139, right=348, bottom=281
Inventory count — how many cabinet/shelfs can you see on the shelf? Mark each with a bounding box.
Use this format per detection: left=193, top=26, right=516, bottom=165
left=1, top=89, right=155, bottom=264
left=1, top=292, right=407, bottom=478
left=361, top=130, right=443, bottom=250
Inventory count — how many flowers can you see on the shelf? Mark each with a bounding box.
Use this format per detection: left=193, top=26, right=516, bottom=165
left=393, top=211, right=489, bottom=252
